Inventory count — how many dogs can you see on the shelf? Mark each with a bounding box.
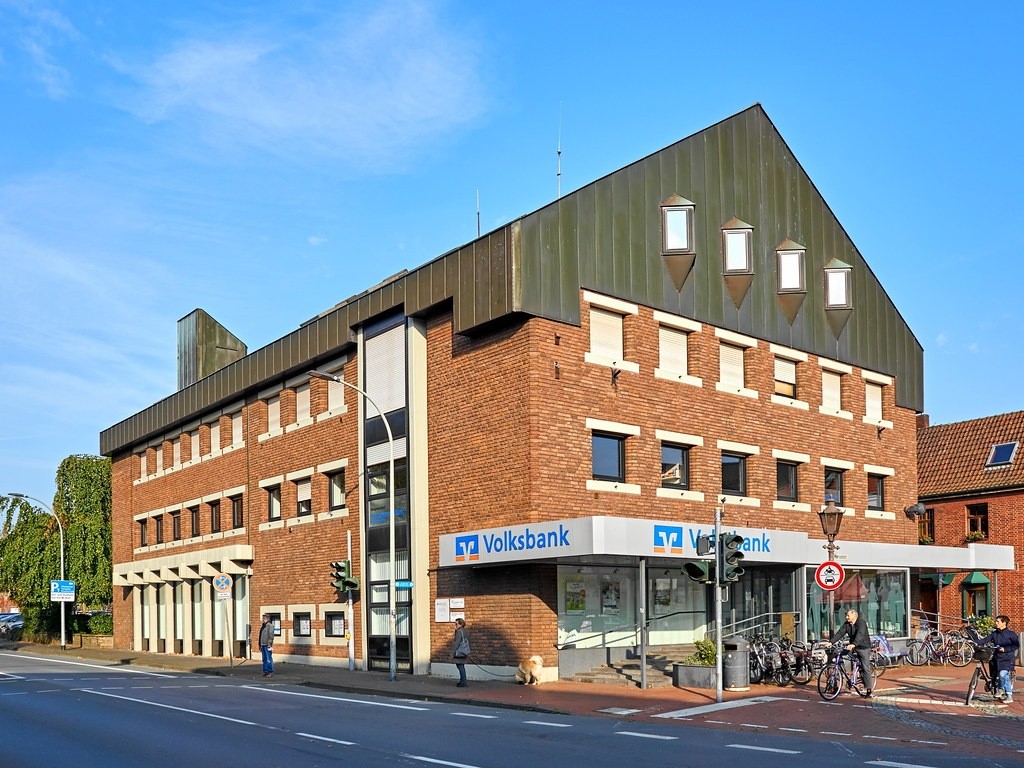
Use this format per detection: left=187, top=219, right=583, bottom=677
left=514, top=655, right=543, bottom=685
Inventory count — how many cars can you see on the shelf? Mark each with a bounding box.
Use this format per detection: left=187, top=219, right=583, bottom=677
left=0, top=613, right=26, bottom=641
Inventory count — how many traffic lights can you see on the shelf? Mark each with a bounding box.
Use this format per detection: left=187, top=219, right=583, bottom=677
left=719, top=534, right=746, bottom=582
left=330, top=560, right=359, bottom=593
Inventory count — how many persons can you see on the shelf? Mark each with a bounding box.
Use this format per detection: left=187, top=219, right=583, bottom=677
left=448, top=618, right=469, bottom=686
left=824, top=609, right=873, bottom=698
left=967, top=615, right=1020, bottom=703
left=258, top=614, right=274, bottom=677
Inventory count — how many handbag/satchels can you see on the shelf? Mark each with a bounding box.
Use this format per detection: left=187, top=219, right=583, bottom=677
left=455, top=638, right=470, bottom=657
left=971, top=644, right=992, bottom=661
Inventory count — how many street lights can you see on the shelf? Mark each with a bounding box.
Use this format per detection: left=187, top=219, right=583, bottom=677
left=817, top=493, right=846, bottom=676
left=306, top=370, right=398, bottom=680
left=7, top=492, right=65, bottom=650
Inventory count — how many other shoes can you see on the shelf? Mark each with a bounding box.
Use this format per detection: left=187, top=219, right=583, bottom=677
left=994, top=688, right=1004, bottom=697
left=865, top=688, right=871, bottom=698
left=1003, top=692, right=1014, bottom=704
left=260, top=672, right=267, bottom=676
left=266, top=674, right=273, bottom=677
left=457, top=681, right=465, bottom=686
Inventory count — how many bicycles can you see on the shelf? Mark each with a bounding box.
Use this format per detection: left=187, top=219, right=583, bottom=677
left=749, top=623, right=1016, bottom=705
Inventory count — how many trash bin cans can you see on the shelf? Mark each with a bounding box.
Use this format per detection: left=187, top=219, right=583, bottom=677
left=723, top=634, right=752, bottom=692
